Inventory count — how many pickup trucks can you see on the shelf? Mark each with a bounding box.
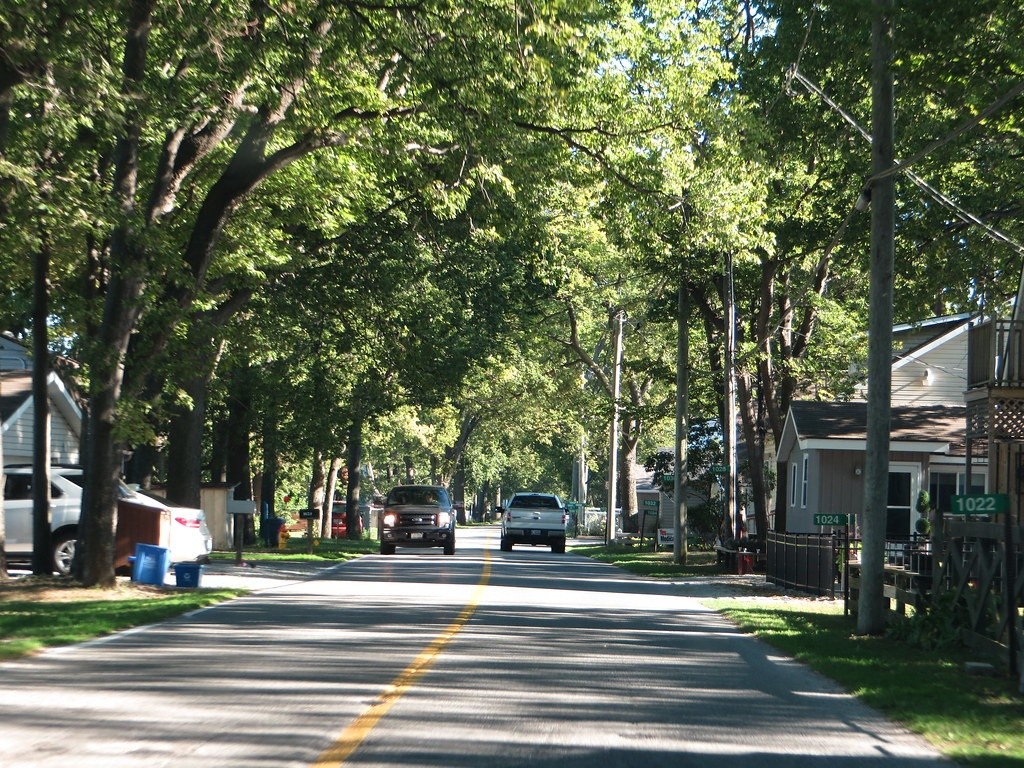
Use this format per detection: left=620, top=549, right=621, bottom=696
left=494, top=492, right=567, bottom=554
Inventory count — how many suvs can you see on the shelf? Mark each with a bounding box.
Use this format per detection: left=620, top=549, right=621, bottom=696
left=4, top=464, right=141, bottom=576
left=379, top=485, right=457, bottom=555
left=326, top=501, right=363, bottom=537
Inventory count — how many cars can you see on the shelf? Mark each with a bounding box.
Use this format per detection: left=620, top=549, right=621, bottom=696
left=126, top=486, right=212, bottom=566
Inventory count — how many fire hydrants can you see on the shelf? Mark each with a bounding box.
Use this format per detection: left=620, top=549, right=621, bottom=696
left=278, top=525, right=290, bottom=549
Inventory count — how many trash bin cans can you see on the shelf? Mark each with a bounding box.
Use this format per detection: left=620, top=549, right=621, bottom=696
left=130, top=543, right=170, bottom=585
left=172, top=560, right=205, bottom=586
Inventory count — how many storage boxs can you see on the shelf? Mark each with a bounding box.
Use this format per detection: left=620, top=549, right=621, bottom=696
left=172, top=563, right=205, bottom=588
left=127, top=555, right=171, bottom=581
